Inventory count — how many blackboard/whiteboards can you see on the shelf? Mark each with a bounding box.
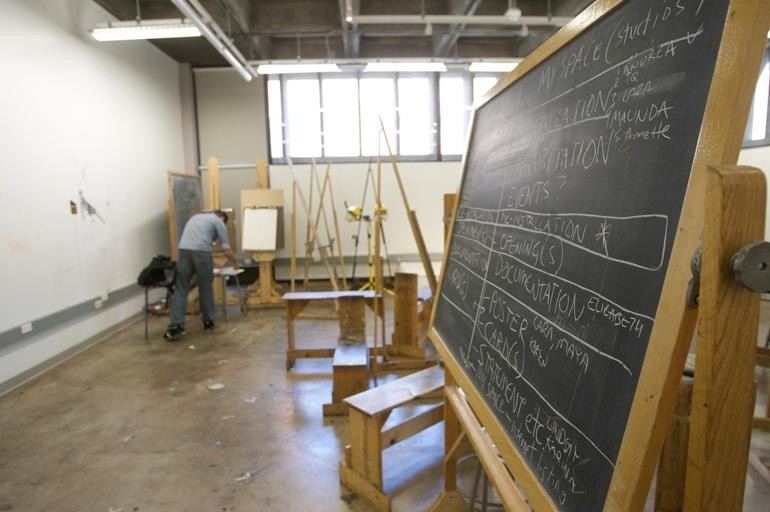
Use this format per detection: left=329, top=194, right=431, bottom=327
left=428, top=2, right=767, bottom=512
left=168, top=170, right=202, bottom=260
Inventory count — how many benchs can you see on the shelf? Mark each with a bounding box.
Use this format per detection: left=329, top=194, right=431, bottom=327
left=279, top=290, right=444, bottom=512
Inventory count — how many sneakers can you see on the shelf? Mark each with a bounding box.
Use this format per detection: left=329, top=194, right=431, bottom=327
left=203, top=319, right=214, bottom=332
left=164, top=326, right=182, bottom=340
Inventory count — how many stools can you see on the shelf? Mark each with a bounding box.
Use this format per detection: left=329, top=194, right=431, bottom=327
left=212, top=267, right=249, bottom=324
left=137, top=261, right=183, bottom=339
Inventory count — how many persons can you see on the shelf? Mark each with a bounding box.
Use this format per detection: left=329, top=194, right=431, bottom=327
left=163, top=210, right=240, bottom=341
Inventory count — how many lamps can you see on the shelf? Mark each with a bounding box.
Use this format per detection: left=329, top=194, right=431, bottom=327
left=90, top=15, right=204, bottom=41
